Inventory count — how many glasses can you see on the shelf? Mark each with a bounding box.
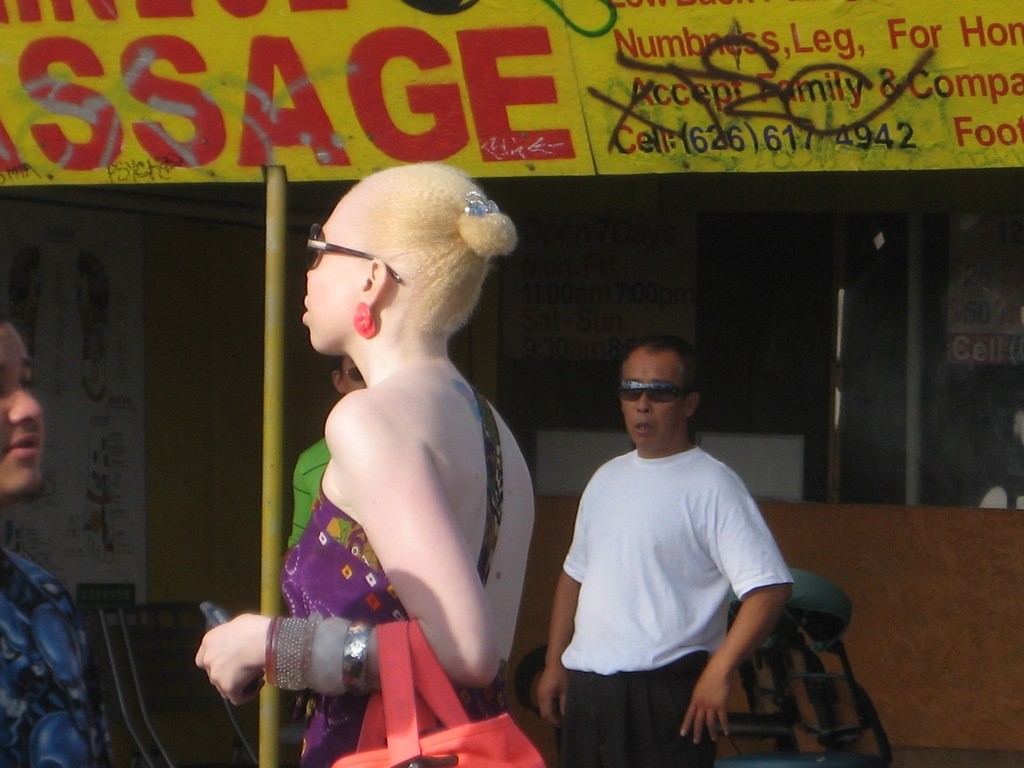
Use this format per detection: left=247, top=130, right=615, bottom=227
left=306, top=223, right=402, bottom=284
left=616, top=379, right=689, bottom=403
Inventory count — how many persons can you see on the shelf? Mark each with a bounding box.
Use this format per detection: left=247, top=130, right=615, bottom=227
left=0, top=315, right=116, bottom=768
left=194, top=161, right=535, bottom=768
left=535, top=333, right=795, bottom=768
left=285, top=353, right=366, bottom=554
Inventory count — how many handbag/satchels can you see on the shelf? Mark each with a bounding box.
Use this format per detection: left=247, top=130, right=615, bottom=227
left=331, top=618, right=545, bottom=768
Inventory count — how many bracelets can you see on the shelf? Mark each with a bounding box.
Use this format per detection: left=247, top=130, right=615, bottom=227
left=264, top=616, right=373, bottom=698
